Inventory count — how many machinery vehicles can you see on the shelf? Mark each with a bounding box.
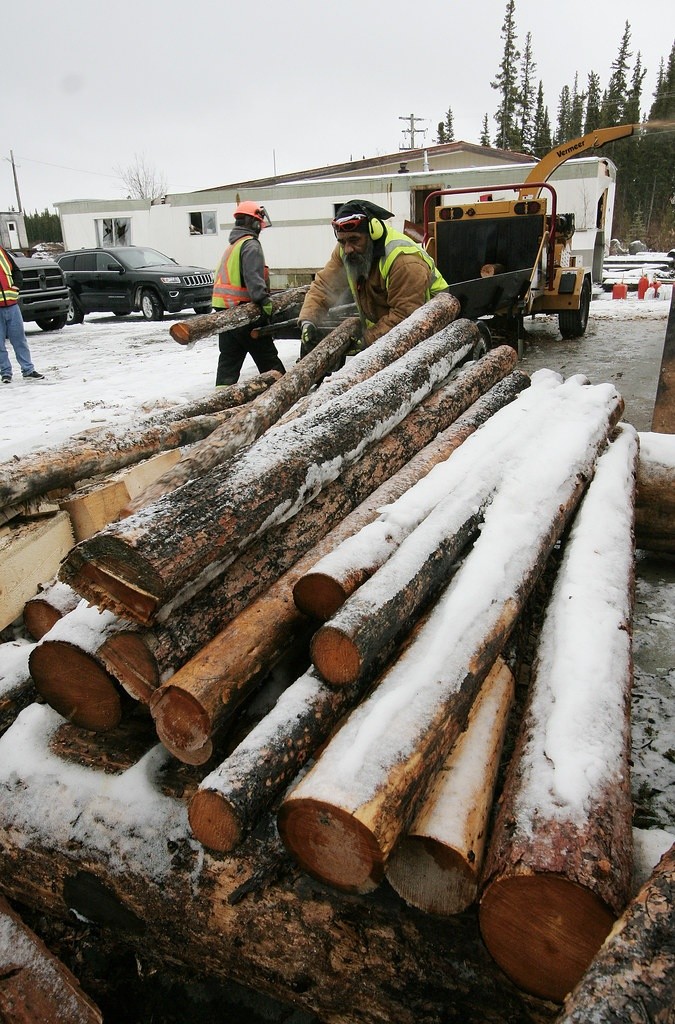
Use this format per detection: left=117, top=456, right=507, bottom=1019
left=422, top=118, right=675, bottom=350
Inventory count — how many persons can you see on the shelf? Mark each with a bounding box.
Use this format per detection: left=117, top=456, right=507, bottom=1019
left=296, top=199, right=450, bottom=356
left=210, top=199, right=289, bottom=387
left=0, top=245, right=45, bottom=383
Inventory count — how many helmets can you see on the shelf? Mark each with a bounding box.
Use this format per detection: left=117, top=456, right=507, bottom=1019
left=234, top=200, right=267, bottom=229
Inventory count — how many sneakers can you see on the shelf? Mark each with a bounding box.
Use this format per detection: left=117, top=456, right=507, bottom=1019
left=2, top=375, right=11, bottom=383
left=23, top=371, right=44, bottom=380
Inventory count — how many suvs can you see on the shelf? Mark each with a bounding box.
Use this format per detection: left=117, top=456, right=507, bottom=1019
left=7, top=255, right=72, bottom=331
left=56, top=244, right=215, bottom=320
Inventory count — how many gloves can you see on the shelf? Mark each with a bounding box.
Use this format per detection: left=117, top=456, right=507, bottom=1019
left=340, top=330, right=366, bottom=358
left=302, top=323, right=318, bottom=354
left=263, top=300, right=281, bottom=317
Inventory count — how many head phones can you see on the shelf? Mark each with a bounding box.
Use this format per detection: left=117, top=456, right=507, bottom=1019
left=235, top=218, right=260, bottom=233
left=333, top=202, right=384, bottom=241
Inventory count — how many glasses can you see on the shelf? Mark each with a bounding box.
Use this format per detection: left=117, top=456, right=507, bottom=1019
left=332, top=216, right=368, bottom=231
